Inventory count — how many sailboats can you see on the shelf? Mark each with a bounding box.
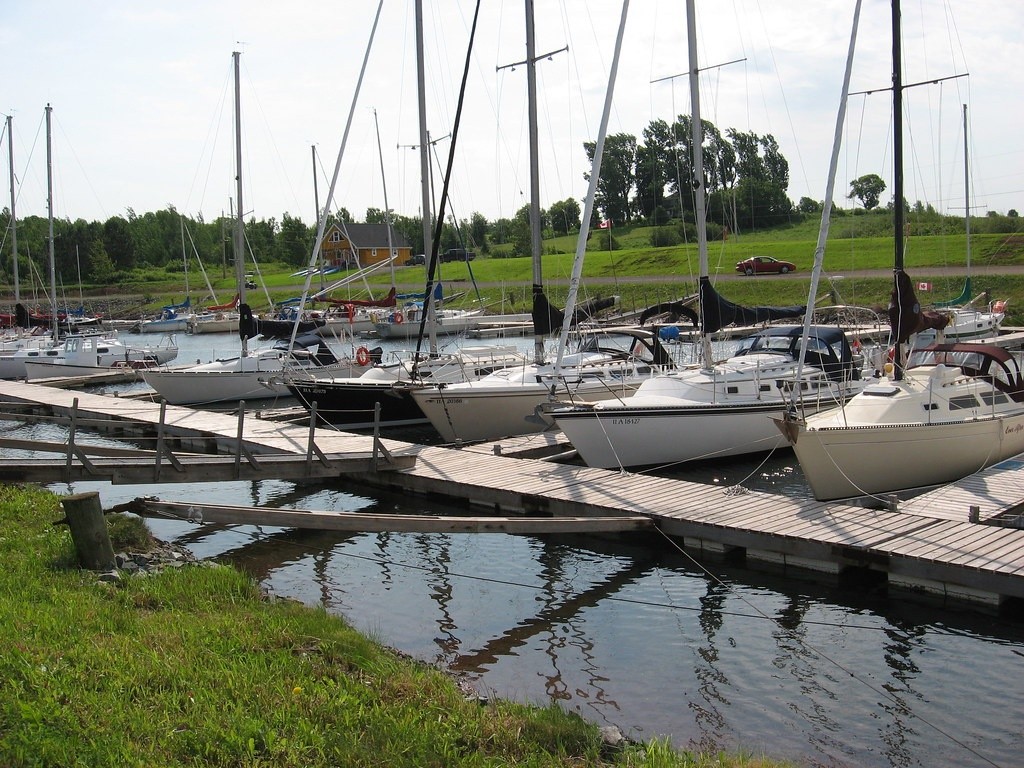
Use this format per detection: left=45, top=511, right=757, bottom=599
left=0, top=0, right=1024, bottom=509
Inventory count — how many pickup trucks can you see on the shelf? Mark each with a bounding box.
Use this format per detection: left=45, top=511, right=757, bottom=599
left=439, top=249, right=476, bottom=262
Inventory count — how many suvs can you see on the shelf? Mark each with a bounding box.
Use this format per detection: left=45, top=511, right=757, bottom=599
left=405, top=254, right=425, bottom=266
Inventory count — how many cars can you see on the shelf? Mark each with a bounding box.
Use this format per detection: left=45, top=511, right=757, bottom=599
left=734, top=256, right=796, bottom=276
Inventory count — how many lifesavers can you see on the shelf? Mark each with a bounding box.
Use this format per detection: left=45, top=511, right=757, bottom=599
left=395, top=313, right=403, bottom=324
left=888, top=345, right=905, bottom=363
left=356, top=347, right=369, bottom=366
